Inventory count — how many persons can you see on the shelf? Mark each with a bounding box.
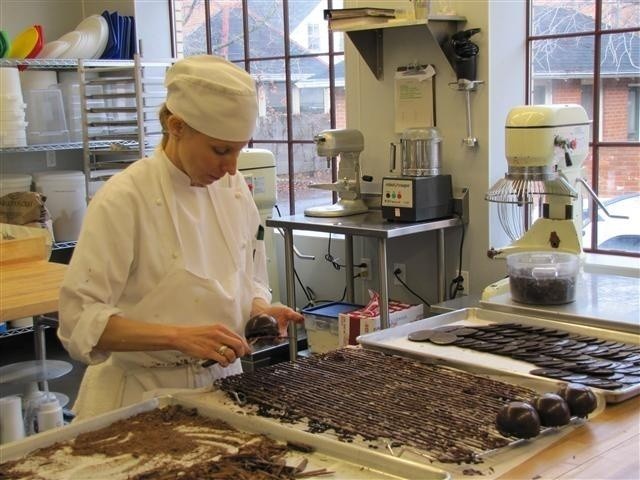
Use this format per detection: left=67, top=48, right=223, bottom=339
left=57, top=55, right=305, bottom=425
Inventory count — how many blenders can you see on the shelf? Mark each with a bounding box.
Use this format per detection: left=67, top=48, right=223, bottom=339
left=482, top=104, right=590, bottom=302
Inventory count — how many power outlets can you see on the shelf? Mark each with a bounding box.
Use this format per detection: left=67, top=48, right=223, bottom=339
left=454, top=269, right=470, bottom=295
left=393, top=263, right=407, bottom=286
left=360, top=258, right=372, bottom=281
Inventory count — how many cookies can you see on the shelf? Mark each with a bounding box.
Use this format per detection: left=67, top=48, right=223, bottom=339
left=408, top=322, right=640, bottom=389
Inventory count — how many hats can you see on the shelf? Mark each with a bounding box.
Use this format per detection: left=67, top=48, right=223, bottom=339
left=165, top=55, right=257, bottom=142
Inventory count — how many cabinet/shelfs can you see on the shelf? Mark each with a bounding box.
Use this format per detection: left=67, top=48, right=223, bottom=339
left=0, top=57, right=144, bottom=339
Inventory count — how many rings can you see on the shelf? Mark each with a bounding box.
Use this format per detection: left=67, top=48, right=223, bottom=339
left=219, top=346, right=228, bottom=355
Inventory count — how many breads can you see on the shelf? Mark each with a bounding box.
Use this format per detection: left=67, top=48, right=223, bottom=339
left=244, top=313, right=278, bottom=339
left=496, top=383, right=597, bottom=438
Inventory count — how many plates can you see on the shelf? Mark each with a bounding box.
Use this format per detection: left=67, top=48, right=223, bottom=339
left=9, top=14, right=110, bottom=60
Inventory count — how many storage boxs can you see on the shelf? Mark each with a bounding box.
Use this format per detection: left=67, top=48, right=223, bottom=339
left=60, top=81, right=109, bottom=141
left=84, top=169, right=130, bottom=204
left=83, top=75, right=139, bottom=139
left=32, top=167, right=88, bottom=242
left=22, top=88, right=69, bottom=146
left=301, top=300, right=423, bottom=355
left=504, top=250, right=579, bottom=306
left=0, top=173, right=34, bottom=201
